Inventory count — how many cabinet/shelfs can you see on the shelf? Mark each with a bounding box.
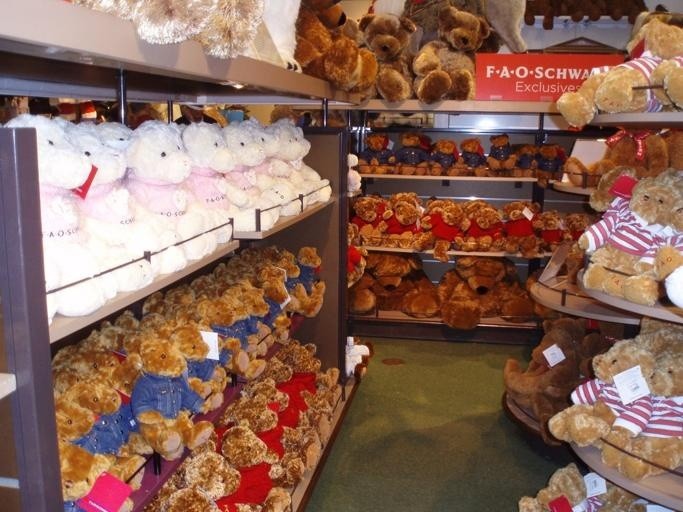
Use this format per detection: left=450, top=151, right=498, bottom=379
left=0, top=0, right=367, bottom=511
left=301, top=89, right=614, bottom=348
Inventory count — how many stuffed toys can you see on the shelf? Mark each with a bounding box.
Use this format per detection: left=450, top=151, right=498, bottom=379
left=0, top=1, right=682, bottom=512
